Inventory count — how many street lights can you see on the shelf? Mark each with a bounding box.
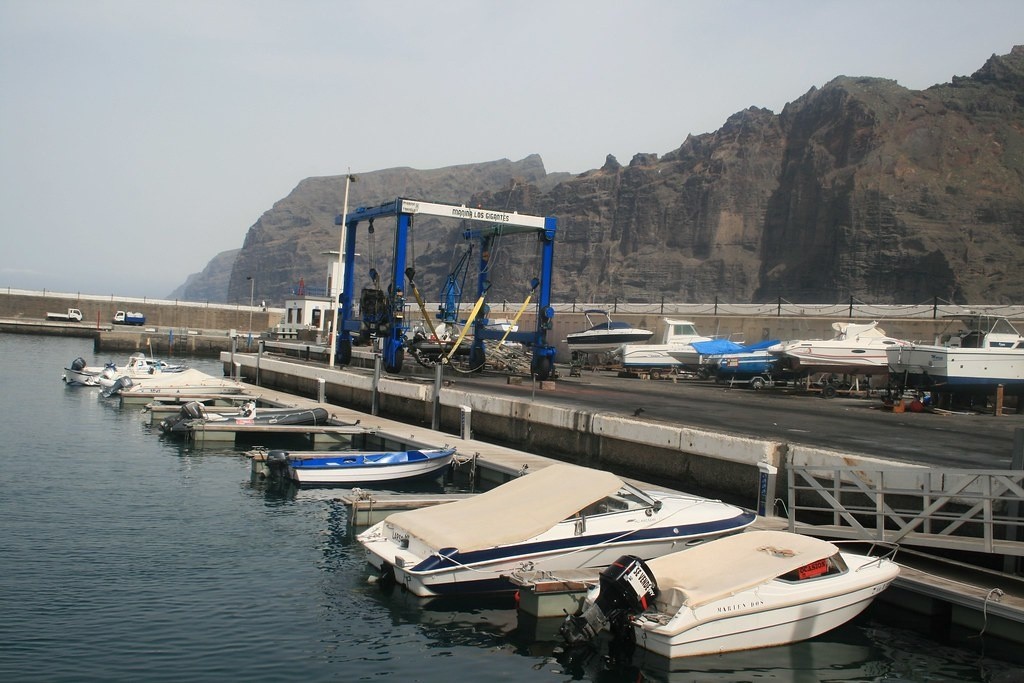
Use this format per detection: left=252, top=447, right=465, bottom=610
left=329, top=166, right=356, bottom=367
left=247, top=277, right=254, bottom=352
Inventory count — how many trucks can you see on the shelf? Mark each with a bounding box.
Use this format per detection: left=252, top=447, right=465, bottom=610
left=46, top=308, right=82, bottom=322
left=112, top=311, right=145, bottom=326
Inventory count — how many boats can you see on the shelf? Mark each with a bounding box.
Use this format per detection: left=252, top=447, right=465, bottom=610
left=567, top=309, right=655, bottom=353
left=885, top=310, right=1024, bottom=388
left=356, top=463, right=757, bottom=596
left=768, top=309, right=914, bottom=375
left=259, top=443, right=457, bottom=490
left=457, top=318, right=519, bottom=336
left=64, top=338, right=247, bottom=404
left=552, top=530, right=901, bottom=659
left=621, top=317, right=818, bottom=380
left=160, top=401, right=328, bottom=436
